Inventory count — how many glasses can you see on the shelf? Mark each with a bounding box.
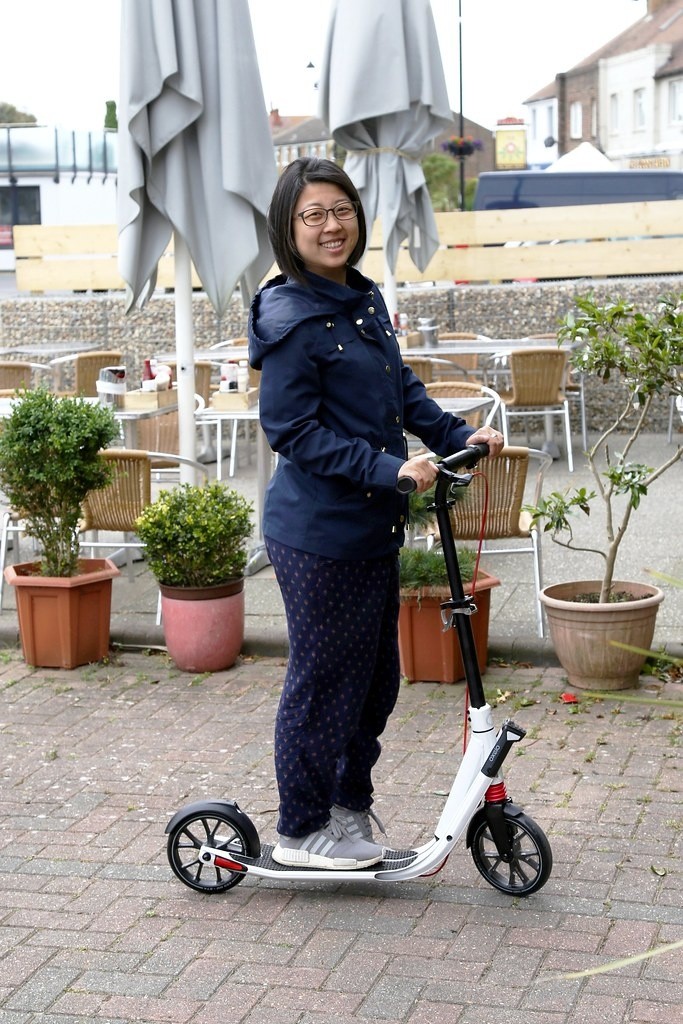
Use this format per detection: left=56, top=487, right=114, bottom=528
left=293, top=202, right=358, bottom=226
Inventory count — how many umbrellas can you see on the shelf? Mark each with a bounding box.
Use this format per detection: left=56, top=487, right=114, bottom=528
left=113, top=1, right=281, bottom=484
left=0, top=101, right=48, bottom=175
left=315, top=0, right=454, bottom=332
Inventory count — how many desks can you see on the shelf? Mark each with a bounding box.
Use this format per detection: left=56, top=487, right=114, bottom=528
left=8, top=342, right=107, bottom=396
left=154, top=349, right=260, bottom=464
left=393, top=334, right=586, bottom=458
left=1, top=396, right=178, bottom=569
left=192, top=393, right=497, bottom=578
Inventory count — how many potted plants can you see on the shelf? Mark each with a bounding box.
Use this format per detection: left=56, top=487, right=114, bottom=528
left=393, top=455, right=504, bottom=682
left=516, top=288, right=683, bottom=687
left=0, top=380, right=118, bottom=671
left=132, top=477, right=255, bottom=671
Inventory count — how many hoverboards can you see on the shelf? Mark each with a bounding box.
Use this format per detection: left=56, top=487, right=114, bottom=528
left=162, top=440, right=555, bottom=898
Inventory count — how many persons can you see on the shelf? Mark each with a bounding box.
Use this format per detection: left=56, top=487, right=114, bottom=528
left=248, top=157, right=504, bottom=869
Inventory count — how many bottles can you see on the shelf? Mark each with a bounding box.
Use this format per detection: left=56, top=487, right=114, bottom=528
left=143, top=358, right=172, bottom=392
left=391, top=312, right=410, bottom=336
left=219, top=360, right=249, bottom=393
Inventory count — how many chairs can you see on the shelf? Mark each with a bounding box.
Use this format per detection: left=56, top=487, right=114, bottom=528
left=0, top=338, right=589, bottom=474
left=77, top=447, right=214, bottom=624
left=0, top=455, right=99, bottom=615
left=403, top=447, right=553, bottom=640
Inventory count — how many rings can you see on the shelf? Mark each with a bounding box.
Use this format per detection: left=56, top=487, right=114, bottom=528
left=491, top=434, right=497, bottom=439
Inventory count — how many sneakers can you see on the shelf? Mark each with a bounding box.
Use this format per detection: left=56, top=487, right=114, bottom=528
left=330, top=804, right=388, bottom=843
left=272, top=819, right=386, bottom=870
